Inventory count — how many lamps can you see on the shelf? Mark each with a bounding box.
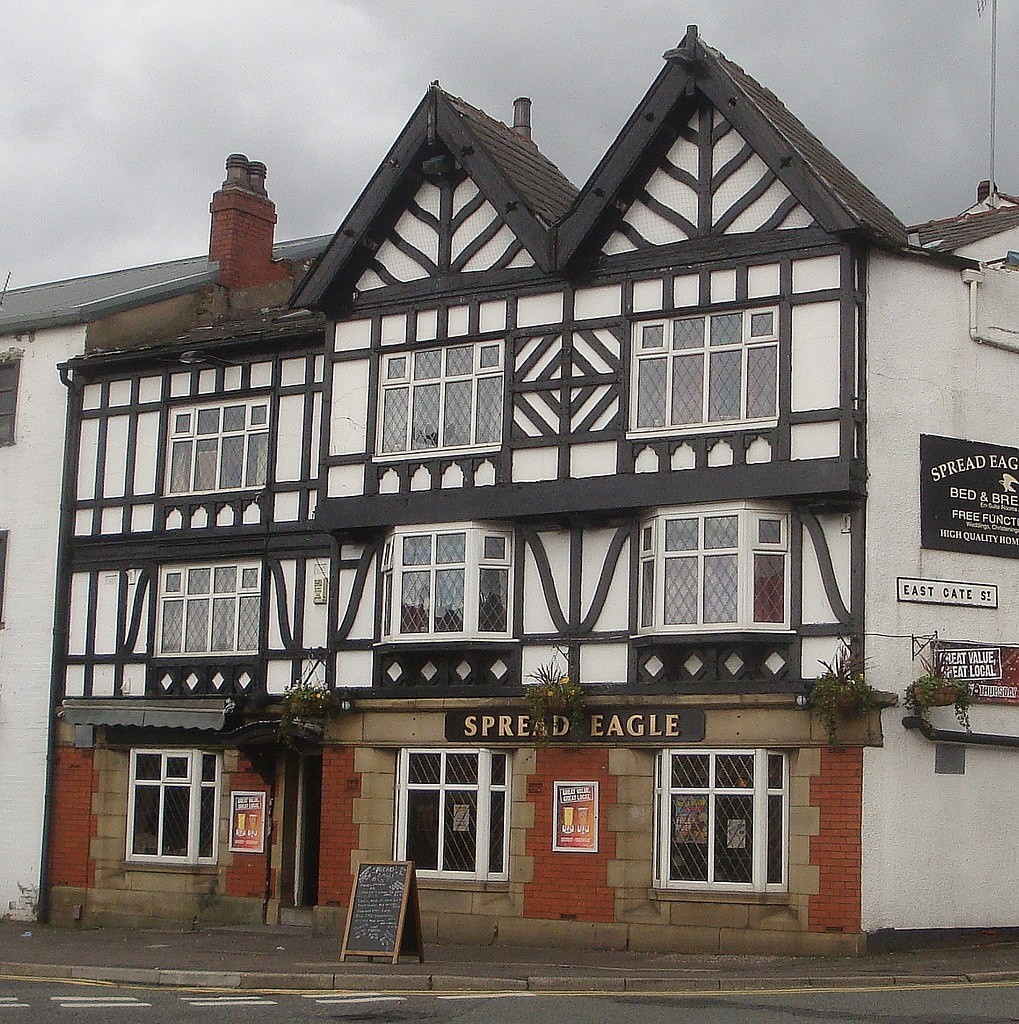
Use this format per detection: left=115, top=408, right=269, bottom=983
left=337, top=684, right=356, bottom=715
left=793, top=682, right=810, bottom=711
left=986, top=249, right=1019, bottom=271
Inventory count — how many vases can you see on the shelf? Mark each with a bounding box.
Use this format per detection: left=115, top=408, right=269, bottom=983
left=287, top=694, right=331, bottom=712
left=532, top=692, right=575, bottom=709
left=821, top=686, right=856, bottom=708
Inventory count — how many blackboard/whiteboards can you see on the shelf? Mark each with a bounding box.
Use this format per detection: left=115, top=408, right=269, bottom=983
left=342, top=861, right=425, bottom=957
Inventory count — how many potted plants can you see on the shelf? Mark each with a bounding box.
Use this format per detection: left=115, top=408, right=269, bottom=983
left=900, top=651, right=977, bottom=741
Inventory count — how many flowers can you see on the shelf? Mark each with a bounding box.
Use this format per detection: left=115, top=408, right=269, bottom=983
left=807, top=647, right=883, bottom=753
left=520, top=664, right=589, bottom=762
left=278, top=677, right=333, bottom=733
